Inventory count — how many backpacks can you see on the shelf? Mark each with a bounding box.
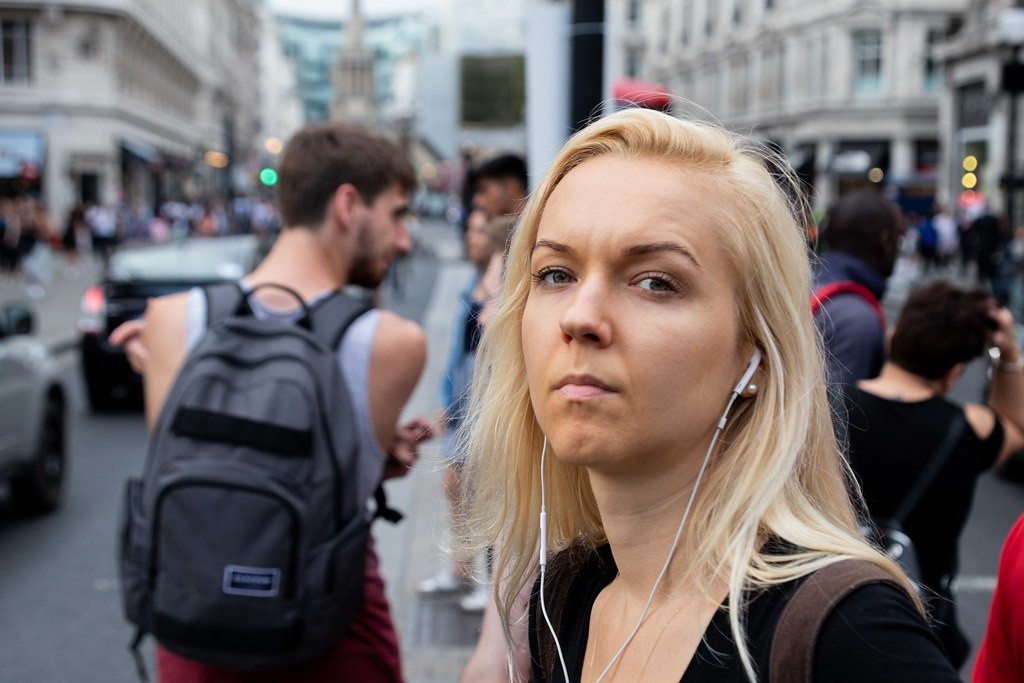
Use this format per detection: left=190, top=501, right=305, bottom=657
left=119, top=280, right=375, bottom=671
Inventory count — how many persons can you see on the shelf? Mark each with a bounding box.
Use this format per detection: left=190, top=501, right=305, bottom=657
left=110, top=126, right=435, bottom=683
left=806, top=188, right=1023, bottom=683
left=416, top=154, right=529, bottom=609
left=448, top=107, right=960, bottom=683
left=0, top=191, right=274, bottom=283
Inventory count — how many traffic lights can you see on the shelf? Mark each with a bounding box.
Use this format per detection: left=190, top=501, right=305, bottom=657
left=258, top=166, right=279, bottom=190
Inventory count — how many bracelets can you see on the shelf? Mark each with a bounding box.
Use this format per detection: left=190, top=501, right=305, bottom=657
left=993, top=357, right=1023, bottom=373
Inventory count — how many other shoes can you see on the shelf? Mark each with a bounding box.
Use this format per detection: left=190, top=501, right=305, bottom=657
left=416, top=569, right=465, bottom=592
left=460, top=585, right=491, bottom=613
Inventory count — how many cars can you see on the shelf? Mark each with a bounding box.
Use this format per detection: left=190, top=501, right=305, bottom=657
left=77, top=235, right=267, bottom=417
left=0, top=298, right=73, bottom=528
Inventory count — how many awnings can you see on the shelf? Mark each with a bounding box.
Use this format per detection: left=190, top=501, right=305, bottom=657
left=0, top=129, right=48, bottom=178
left=123, top=132, right=192, bottom=172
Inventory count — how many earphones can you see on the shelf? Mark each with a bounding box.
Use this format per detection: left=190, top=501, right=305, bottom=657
left=733, top=345, right=760, bottom=396
